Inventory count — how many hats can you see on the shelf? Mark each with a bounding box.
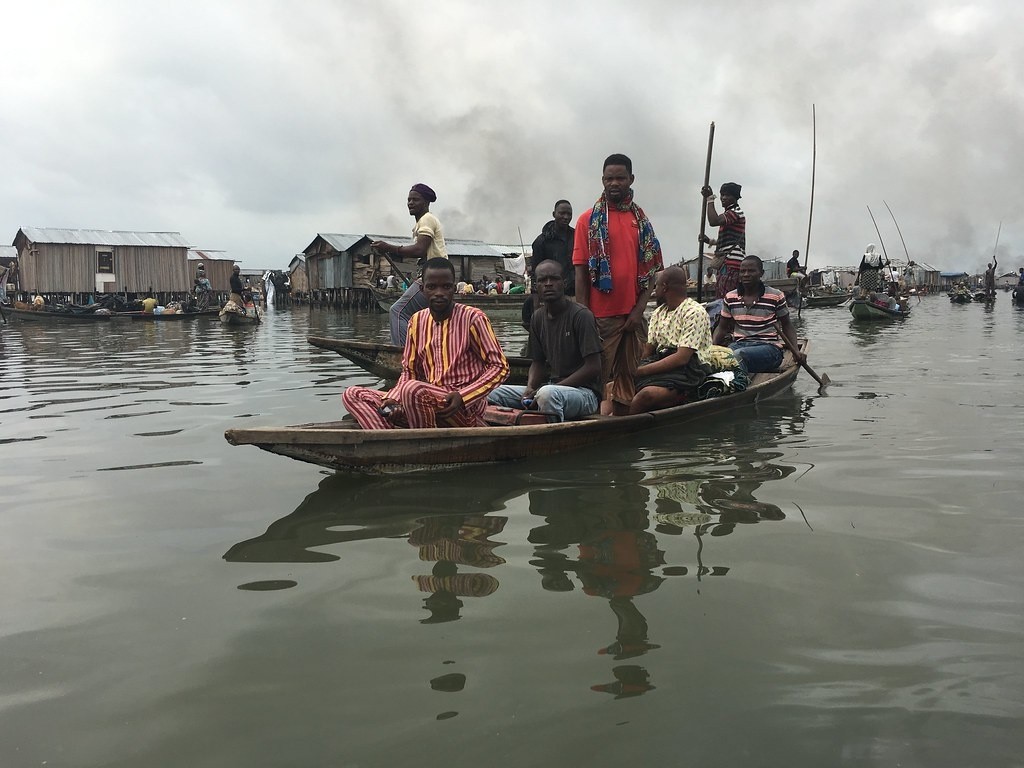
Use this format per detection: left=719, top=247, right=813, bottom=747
left=197, top=262, right=204, bottom=268
left=410, top=183, right=437, bottom=202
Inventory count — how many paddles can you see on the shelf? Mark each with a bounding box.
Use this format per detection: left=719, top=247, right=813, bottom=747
left=248, top=281, right=260, bottom=324
left=991, top=221, right=1002, bottom=269
left=882, top=200, right=921, bottom=304
left=798, top=102, right=817, bottom=315
left=516, top=225, right=528, bottom=271
left=696, top=121, right=718, bottom=304
left=766, top=318, right=831, bottom=394
left=865, top=203, right=904, bottom=321
left=380, top=250, right=412, bottom=287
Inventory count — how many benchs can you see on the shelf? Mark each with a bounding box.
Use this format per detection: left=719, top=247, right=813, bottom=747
left=484, top=404, right=547, bottom=426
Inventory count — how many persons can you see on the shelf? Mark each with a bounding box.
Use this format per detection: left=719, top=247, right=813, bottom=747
left=710, top=255, right=808, bottom=373
left=831, top=280, right=861, bottom=298
left=856, top=243, right=891, bottom=301
left=388, top=183, right=449, bottom=345
left=454, top=273, right=516, bottom=295
left=984, top=255, right=997, bottom=298
left=189, top=262, right=212, bottom=311
left=229, top=264, right=252, bottom=312
left=786, top=249, right=810, bottom=292
left=342, top=257, right=510, bottom=430
left=486, top=154, right=712, bottom=423
left=380, top=270, right=412, bottom=293
left=889, top=260, right=915, bottom=294
left=949, top=281, right=969, bottom=294
left=1012, top=267, right=1024, bottom=298
left=142, top=293, right=157, bottom=313
left=698, top=181, right=746, bottom=298
left=704, top=267, right=717, bottom=300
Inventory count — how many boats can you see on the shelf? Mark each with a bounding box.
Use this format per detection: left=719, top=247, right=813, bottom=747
left=306, top=335, right=532, bottom=388
left=686, top=276, right=811, bottom=307
left=367, top=283, right=531, bottom=320
left=849, top=298, right=911, bottom=320
left=133, top=307, right=224, bottom=321
left=0, top=301, right=145, bottom=324
left=948, top=294, right=971, bottom=302
left=219, top=303, right=261, bottom=324
left=980, top=297, right=997, bottom=303
left=805, top=292, right=855, bottom=306
left=911, top=290, right=926, bottom=296
left=224, top=338, right=809, bottom=472
left=899, top=292, right=912, bottom=298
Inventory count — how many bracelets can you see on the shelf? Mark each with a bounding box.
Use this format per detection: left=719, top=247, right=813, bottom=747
left=706, top=194, right=717, bottom=204
left=397, top=245, right=404, bottom=254
left=708, top=238, right=717, bottom=248
